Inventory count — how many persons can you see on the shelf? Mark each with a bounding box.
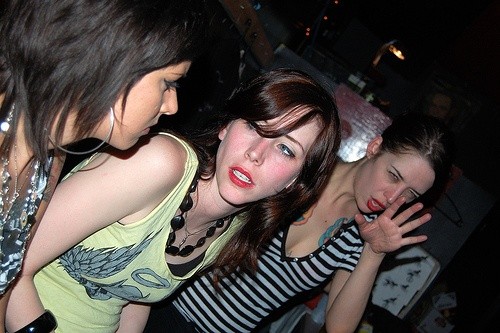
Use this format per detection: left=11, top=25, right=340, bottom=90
left=4, top=66, right=342, bottom=333
left=374, top=39, right=457, bottom=119
left=0, top=0, right=200, bottom=333
left=141, top=113, right=458, bottom=333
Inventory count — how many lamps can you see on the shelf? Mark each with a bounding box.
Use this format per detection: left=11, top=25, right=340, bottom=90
left=373, top=40, right=408, bottom=68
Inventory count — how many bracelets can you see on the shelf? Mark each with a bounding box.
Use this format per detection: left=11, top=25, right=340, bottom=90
left=12, top=310, right=58, bottom=333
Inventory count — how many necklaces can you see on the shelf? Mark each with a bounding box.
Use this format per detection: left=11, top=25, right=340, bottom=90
left=166, top=160, right=234, bottom=256
left=3, top=100, right=37, bottom=219
left=178, top=211, right=217, bottom=248
